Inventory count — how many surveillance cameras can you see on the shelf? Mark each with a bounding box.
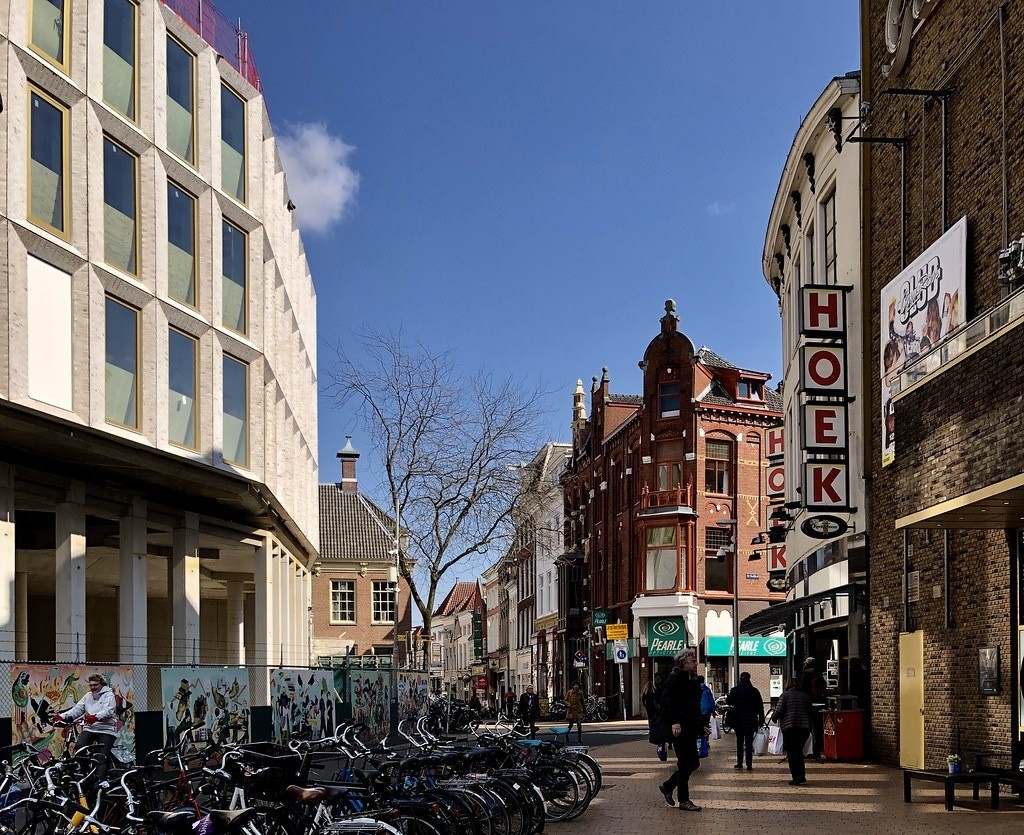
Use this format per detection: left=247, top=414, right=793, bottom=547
left=716, top=549, right=727, bottom=563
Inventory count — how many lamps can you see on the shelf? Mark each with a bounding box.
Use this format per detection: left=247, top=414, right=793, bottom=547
left=750, top=527, right=795, bottom=544
left=748, top=545, right=785, bottom=561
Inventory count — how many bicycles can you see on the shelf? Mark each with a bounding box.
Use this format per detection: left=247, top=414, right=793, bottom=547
left=0, top=688, right=611, bottom=835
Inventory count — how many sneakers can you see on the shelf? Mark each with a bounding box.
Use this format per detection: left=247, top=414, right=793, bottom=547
left=659, top=783, right=675, bottom=806
left=679, top=801, right=702, bottom=811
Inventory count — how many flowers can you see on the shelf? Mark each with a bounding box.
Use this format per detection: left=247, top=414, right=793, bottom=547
left=946, top=754, right=961, bottom=763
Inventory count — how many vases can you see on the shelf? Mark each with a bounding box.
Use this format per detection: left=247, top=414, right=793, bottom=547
left=948, top=762, right=959, bottom=774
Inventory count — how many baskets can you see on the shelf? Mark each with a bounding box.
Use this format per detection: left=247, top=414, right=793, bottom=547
left=224, top=741, right=313, bottom=804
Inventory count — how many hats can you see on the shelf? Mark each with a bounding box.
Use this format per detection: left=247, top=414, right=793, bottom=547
left=803, top=657, right=816, bottom=664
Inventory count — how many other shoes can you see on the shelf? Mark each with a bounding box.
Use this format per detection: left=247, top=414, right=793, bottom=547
left=668, top=746, right=674, bottom=751
left=806, top=753, right=821, bottom=759
left=789, top=778, right=808, bottom=786
left=734, top=764, right=743, bottom=768
left=747, top=767, right=753, bottom=771
left=565, top=735, right=570, bottom=743
left=577, top=738, right=583, bottom=744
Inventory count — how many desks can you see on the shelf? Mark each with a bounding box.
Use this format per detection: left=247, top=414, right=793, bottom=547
left=902, top=768, right=1001, bottom=814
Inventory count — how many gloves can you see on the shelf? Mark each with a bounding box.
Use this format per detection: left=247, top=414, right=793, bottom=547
left=770, top=715, right=779, bottom=723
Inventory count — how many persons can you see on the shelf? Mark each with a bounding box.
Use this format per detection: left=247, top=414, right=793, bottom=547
left=563, top=680, right=588, bottom=745
left=53, top=674, right=118, bottom=782
left=642, top=675, right=720, bottom=762
left=799, top=656, right=827, bottom=752
left=660, top=648, right=711, bottom=811
left=725, top=672, right=765, bottom=771
left=772, top=676, right=813, bottom=786
left=504, top=687, right=517, bottom=719
left=516, top=684, right=541, bottom=740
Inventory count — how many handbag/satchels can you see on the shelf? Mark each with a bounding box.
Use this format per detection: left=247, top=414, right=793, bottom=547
left=565, top=709, right=568, bottom=718
left=514, top=712, right=526, bottom=734
left=767, top=719, right=784, bottom=755
left=724, top=708, right=734, bottom=727
left=711, top=718, right=721, bottom=740
left=657, top=743, right=668, bottom=760
left=99, top=691, right=132, bottom=715
left=802, top=733, right=813, bottom=757
left=754, top=727, right=767, bottom=756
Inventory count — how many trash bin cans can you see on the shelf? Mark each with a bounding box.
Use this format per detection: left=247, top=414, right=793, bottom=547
left=822, top=695, right=863, bottom=761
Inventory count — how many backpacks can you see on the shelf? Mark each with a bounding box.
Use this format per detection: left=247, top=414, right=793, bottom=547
left=805, top=671, right=826, bottom=703
left=650, top=671, right=700, bottom=732
